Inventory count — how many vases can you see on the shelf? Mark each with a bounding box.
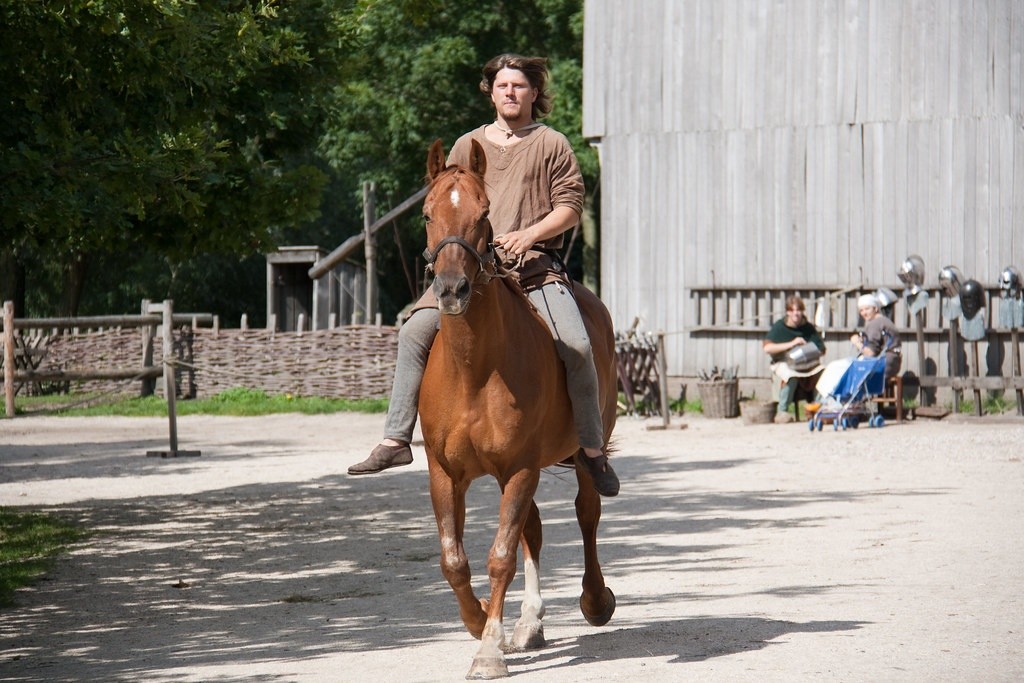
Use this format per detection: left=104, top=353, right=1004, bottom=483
left=740, top=399, right=779, bottom=425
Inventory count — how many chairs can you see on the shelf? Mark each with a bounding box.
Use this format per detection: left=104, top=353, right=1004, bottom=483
left=867, top=376, right=901, bottom=424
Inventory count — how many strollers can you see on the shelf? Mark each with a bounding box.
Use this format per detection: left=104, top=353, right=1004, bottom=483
left=807, top=329, right=894, bottom=431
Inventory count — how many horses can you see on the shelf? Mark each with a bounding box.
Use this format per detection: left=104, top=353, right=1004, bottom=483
left=415, top=136, right=620, bottom=679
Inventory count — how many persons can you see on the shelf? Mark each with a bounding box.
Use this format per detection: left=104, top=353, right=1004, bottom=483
left=762, top=295, right=826, bottom=424
left=844, top=292, right=902, bottom=414
left=346, top=54, right=620, bottom=498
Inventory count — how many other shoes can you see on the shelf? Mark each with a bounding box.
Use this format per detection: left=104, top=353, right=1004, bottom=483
left=774, top=413, right=793, bottom=423
left=803, top=402, right=820, bottom=413
left=347, top=444, right=413, bottom=475
left=578, top=447, right=620, bottom=496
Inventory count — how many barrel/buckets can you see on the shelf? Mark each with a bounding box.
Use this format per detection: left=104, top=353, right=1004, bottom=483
left=785, top=342, right=820, bottom=370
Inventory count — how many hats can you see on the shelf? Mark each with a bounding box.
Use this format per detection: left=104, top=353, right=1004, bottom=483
left=858, top=294, right=881, bottom=310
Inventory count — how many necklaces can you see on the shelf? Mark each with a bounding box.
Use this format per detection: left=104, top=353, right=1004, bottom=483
left=493, top=120, right=545, bottom=139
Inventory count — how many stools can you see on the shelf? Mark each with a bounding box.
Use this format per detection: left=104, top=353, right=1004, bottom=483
left=771, top=375, right=814, bottom=421
